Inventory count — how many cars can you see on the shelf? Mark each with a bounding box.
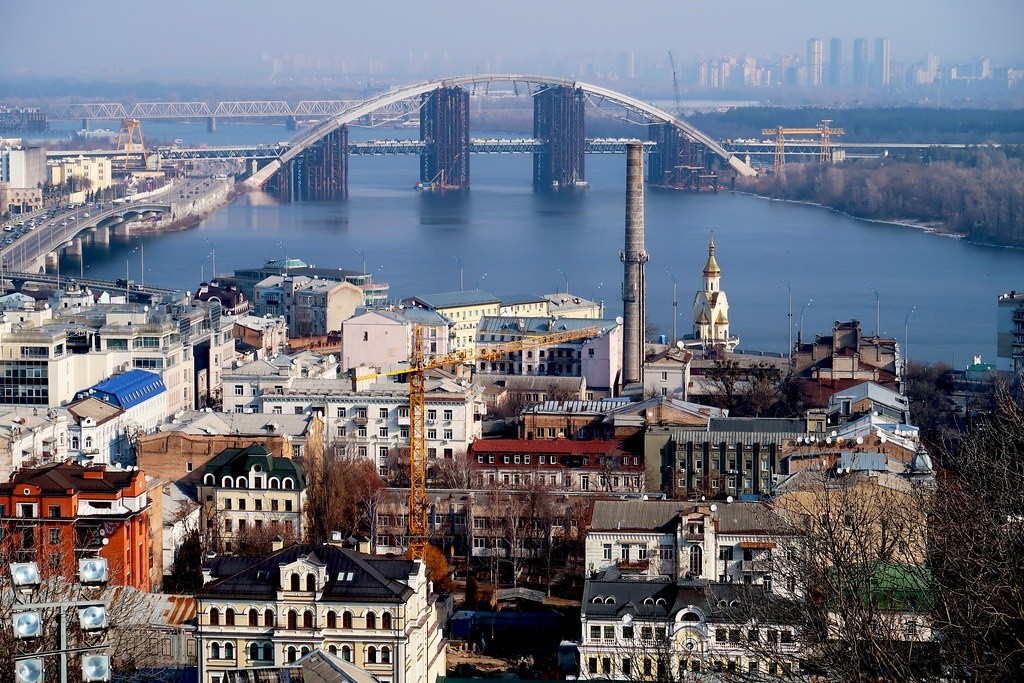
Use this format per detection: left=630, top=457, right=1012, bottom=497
left=65, top=276, right=75, bottom=282
left=0, top=200, right=162, bottom=251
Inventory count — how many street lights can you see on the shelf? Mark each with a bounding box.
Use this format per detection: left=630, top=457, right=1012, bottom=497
left=557, top=268, right=568, bottom=297
left=477, top=273, right=488, bottom=291
left=201, top=252, right=212, bottom=284
left=132, top=233, right=144, bottom=284
left=126, top=246, right=138, bottom=303
left=357, top=248, right=383, bottom=307
left=592, top=282, right=603, bottom=319
left=452, top=257, right=463, bottom=293
left=665, top=266, right=677, bottom=343
left=869, top=286, right=880, bottom=336
left=203, top=237, right=216, bottom=278
left=800, top=296, right=812, bottom=345
left=780, top=279, right=791, bottom=359
left=905, top=304, right=918, bottom=372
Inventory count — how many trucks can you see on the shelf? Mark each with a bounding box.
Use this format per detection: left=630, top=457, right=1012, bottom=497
left=116, top=278, right=134, bottom=289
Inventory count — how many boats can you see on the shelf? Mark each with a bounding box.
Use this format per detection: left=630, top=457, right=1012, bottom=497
left=549, top=167, right=588, bottom=188
left=413, top=169, right=460, bottom=191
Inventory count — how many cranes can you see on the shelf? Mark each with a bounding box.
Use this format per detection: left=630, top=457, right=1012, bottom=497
left=350, top=324, right=598, bottom=566
left=669, top=50, right=680, bottom=114
